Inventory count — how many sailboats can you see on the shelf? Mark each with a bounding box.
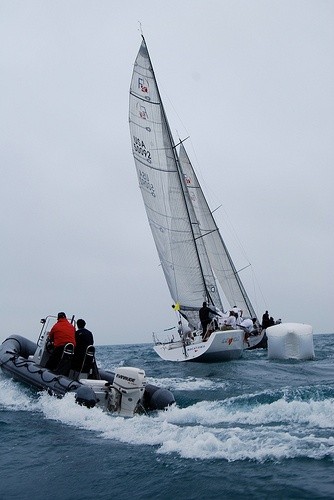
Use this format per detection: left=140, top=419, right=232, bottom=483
left=119, top=19, right=248, bottom=364
left=163, top=126, right=275, bottom=351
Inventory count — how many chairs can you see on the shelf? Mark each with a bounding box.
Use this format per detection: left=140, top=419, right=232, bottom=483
left=52, top=344, right=74, bottom=377
left=79, top=345, right=99, bottom=377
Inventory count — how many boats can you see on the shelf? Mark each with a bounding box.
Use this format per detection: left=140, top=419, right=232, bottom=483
left=0, top=311, right=175, bottom=422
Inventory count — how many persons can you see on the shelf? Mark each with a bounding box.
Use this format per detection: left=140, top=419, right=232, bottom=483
left=270, top=317, right=275, bottom=326
left=276, top=319, right=282, bottom=324
left=48, top=312, right=76, bottom=365
left=199, top=302, right=221, bottom=338
left=224, top=311, right=238, bottom=330
left=232, top=306, right=243, bottom=317
left=240, top=318, right=256, bottom=339
left=76, top=319, right=94, bottom=367
left=177, top=321, right=193, bottom=342
left=262, top=310, right=270, bottom=328
